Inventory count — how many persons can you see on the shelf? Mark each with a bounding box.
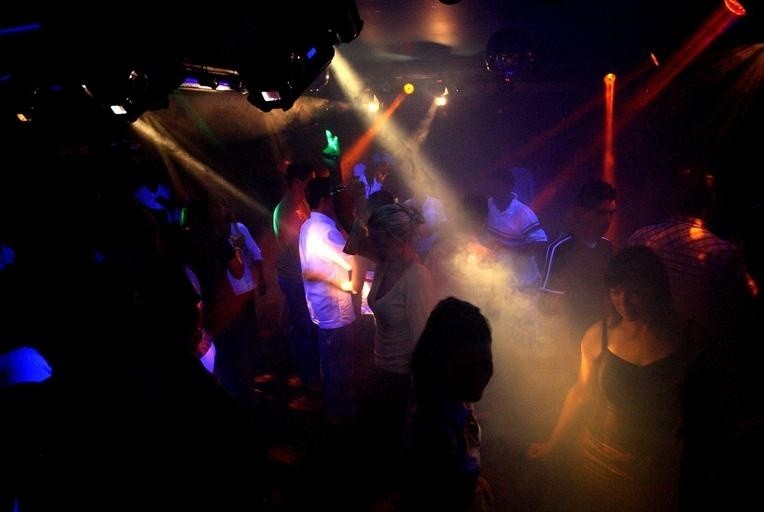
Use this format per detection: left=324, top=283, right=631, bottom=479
left=2, top=145, right=764, bottom=511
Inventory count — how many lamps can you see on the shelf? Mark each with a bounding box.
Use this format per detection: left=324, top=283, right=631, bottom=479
left=35, top=1, right=365, bottom=121
